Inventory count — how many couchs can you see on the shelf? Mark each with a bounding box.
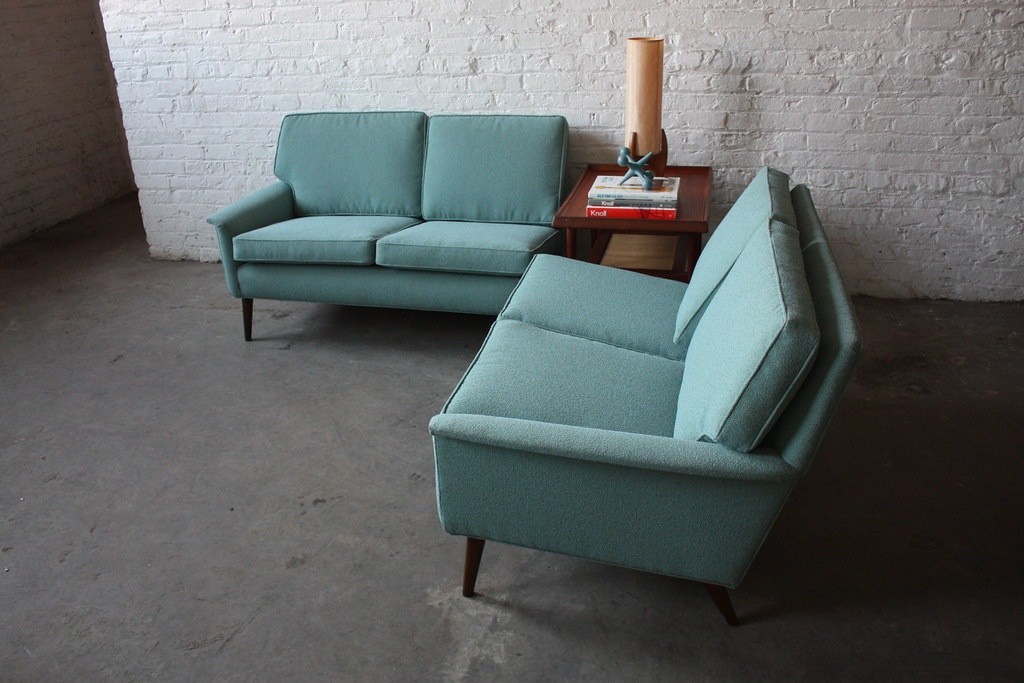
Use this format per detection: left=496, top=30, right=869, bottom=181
left=426, top=165, right=863, bottom=629
left=204, top=110, right=566, bottom=345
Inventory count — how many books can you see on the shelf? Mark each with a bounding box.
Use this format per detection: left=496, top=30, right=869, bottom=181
left=586, top=176, right=680, bottom=207
left=585, top=206, right=676, bottom=220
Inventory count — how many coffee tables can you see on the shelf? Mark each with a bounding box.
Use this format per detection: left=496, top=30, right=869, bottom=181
left=551, top=164, right=712, bottom=284
